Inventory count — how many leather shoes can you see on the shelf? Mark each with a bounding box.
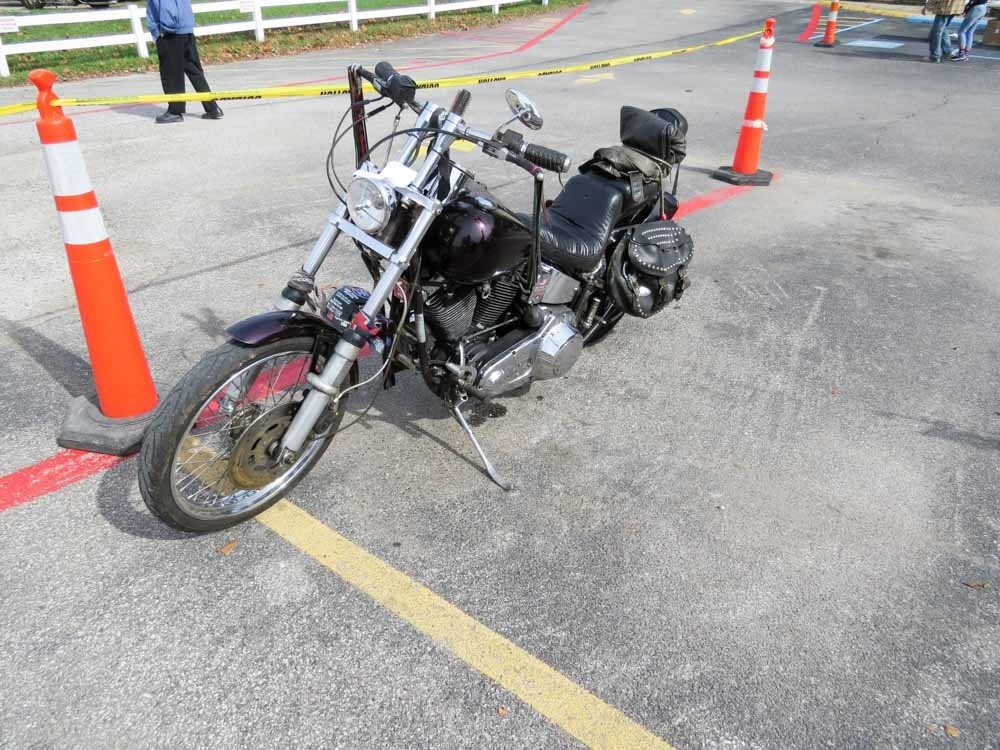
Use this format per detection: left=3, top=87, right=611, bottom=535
left=201, top=107, right=224, bottom=120
left=156, top=111, right=185, bottom=122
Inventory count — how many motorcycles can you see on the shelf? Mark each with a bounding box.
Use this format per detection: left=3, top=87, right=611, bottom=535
left=133, top=57, right=696, bottom=535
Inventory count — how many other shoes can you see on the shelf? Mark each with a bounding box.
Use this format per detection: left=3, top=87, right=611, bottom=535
left=949, top=53, right=966, bottom=60
left=923, top=55, right=941, bottom=64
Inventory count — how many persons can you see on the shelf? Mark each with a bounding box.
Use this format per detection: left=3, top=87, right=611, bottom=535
left=147, top=0, right=224, bottom=123
left=922, top=0, right=965, bottom=64
left=948, top=0, right=986, bottom=60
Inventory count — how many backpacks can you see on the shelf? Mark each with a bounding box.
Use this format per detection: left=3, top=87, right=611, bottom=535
left=607, top=219, right=694, bottom=317
